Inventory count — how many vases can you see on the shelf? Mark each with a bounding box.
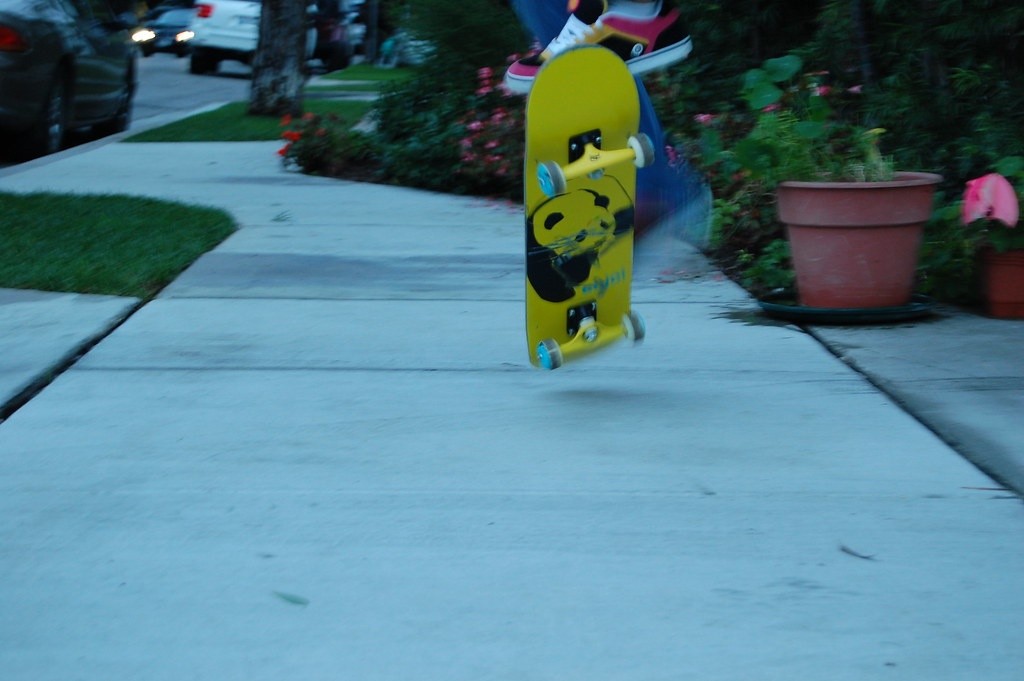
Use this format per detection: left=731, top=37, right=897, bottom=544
left=328, top=161, right=346, bottom=178
left=777, top=172, right=943, bottom=309
left=477, top=173, right=508, bottom=197
left=302, top=155, right=327, bottom=176
left=345, top=164, right=367, bottom=182
left=978, top=247, right=1024, bottom=319
left=367, top=162, right=390, bottom=183
left=440, top=172, right=473, bottom=194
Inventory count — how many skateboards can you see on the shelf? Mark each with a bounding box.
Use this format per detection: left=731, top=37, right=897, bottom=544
left=522, top=45, right=657, bottom=372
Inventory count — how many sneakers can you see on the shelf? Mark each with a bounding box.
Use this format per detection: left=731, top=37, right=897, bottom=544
left=503, top=0, right=691, bottom=95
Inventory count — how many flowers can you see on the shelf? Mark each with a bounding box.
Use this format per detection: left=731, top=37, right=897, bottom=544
left=686, top=54, right=898, bottom=183
left=276, top=66, right=525, bottom=191
left=911, top=156, right=1024, bottom=271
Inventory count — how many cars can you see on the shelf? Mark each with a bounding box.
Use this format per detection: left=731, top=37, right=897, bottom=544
left=133, top=0, right=370, bottom=75
left=0, top=0, right=142, bottom=154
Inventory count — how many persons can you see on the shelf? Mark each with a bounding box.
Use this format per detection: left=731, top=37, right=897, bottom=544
left=505, top=0, right=711, bottom=285
left=314, top=0, right=386, bottom=62
